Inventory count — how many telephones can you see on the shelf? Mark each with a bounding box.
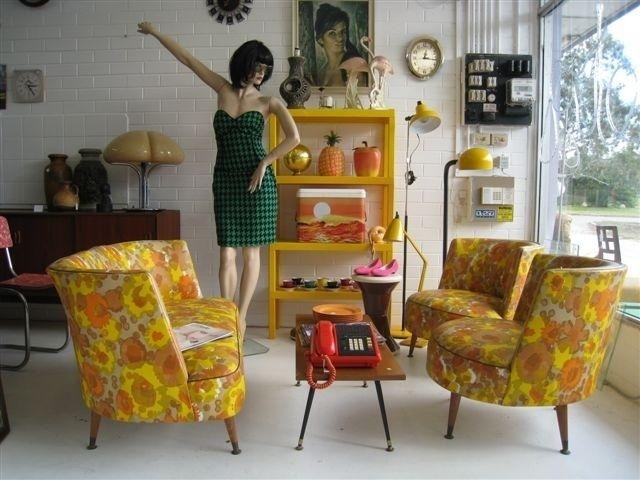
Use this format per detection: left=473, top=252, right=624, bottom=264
left=311, top=321, right=382, bottom=368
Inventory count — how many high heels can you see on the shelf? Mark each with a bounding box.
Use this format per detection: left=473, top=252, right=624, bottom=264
left=354, top=258, right=398, bottom=276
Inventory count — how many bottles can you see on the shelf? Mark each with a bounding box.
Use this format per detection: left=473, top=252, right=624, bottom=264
left=53, top=180, right=80, bottom=212
left=73, top=148, right=109, bottom=207
left=44, top=154, right=72, bottom=212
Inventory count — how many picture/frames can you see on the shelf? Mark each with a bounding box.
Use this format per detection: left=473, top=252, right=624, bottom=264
left=291, top=0, right=375, bottom=94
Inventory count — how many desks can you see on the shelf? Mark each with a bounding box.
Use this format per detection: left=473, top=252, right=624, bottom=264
left=352, top=274, right=401, bottom=356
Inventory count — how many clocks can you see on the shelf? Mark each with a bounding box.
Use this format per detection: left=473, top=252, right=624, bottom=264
left=13, top=69, right=45, bottom=102
left=406, top=35, right=444, bottom=80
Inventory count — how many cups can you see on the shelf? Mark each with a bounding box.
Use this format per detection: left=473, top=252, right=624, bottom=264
left=320, top=96, right=334, bottom=108
left=326, top=280, right=338, bottom=286
left=292, top=277, right=304, bottom=285
left=340, top=277, right=352, bottom=285
left=304, top=280, right=319, bottom=288
left=317, top=277, right=329, bottom=286
left=282, top=280, right=297, bottom=287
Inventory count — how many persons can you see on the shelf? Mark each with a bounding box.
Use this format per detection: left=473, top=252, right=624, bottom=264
left=138, top=21, right=301, bottom=345
left=316, top=3, right=367, bottom=87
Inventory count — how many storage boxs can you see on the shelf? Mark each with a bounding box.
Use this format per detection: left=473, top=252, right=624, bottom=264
left=296, top=188, right=366, bottom=243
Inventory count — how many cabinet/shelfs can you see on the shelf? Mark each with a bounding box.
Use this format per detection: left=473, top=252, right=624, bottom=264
left=269, top=109, right=395, bottom=340
left=0, top=208, right=180, bottom=322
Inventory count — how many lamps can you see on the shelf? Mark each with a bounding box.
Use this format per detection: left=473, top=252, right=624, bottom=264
left=389, top=101, right=441, bottom=336
left=443, top=149, right=493, bottom=262
left=382, top=212, right=427, bottom=348
left=103, top=131, right=185, bottom=210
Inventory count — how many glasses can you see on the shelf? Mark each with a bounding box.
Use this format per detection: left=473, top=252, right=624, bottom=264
left=255, top=64, right=272, bottom=74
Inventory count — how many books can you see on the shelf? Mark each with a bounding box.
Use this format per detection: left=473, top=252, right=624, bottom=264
left=297, top=322, right=386, bottom=350
left=174, top=323, right=235, bottom=353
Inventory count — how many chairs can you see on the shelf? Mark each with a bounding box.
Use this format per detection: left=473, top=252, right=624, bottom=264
left=404, top=238, right=544, bottom=357
left=427, top=254, right=627, bottom=455
left=594, top=225, right=640, bottom=303
left=0, top=216, right=71, bottom=370
left=47, top=239, right=245, bottom=455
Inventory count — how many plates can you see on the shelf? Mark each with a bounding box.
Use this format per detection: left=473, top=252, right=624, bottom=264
left=279, top=286, right=298, bottom=291
left=340, top=285, right=353, bottom=290
left=293, top=285, right=304, bottom=291
left=303, top=286, right=317, bottom=292
left=316, top=284, right=340, bottom=292
left=313, top=304, right=364, bottom=325
